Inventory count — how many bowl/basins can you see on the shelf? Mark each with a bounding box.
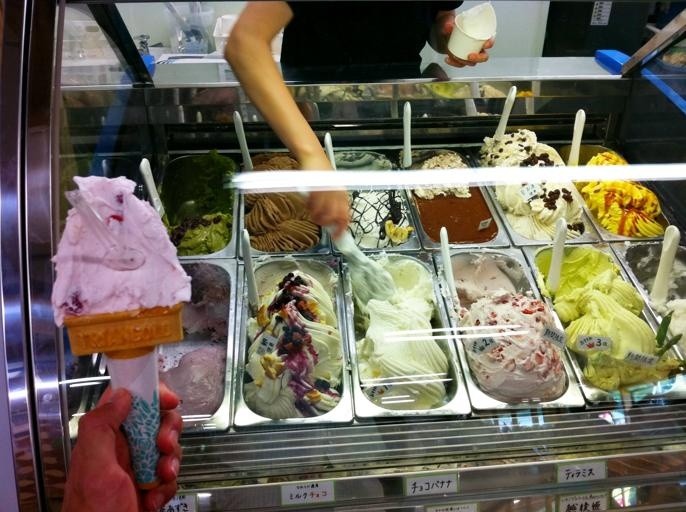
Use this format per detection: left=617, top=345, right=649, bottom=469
left=574, top=183, right=673, bottom=245
left=239, top=151, right=332, bottom=256
left=519, top=241, right=682, bottom=406
left=339, top=250, right=471, bottom=419
left=87, top=256, right=238, bottom=433
left=406, top=155, right=514, bottom=252
left=161, top=153, right=238, bottom=259
left=477, top=146, right=603, bottom=250
left=231, top=254, right=354, bottom=427
left=329, top=159, right=424, bottom=253
left=433, top=247, right=587, bottom=412
left=609, top=240, right=686, bottom=365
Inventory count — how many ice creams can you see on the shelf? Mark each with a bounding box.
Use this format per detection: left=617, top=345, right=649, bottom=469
left=447, top=2, right=497, bottom=61
left=51, top=175, right=192, bottom=490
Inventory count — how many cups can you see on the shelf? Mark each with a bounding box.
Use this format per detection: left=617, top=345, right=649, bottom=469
left=163, top=4, right=217, bottom=60
left=447, top=12, right=497, bottom=63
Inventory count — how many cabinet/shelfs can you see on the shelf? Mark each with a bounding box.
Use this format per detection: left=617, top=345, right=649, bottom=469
left=0, top=0, right=686, bottom=512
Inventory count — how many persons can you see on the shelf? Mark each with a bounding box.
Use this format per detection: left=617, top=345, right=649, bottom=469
left=56, top=378, right=184, bottom=512
left=224, top=0, right=496, bottom=236
